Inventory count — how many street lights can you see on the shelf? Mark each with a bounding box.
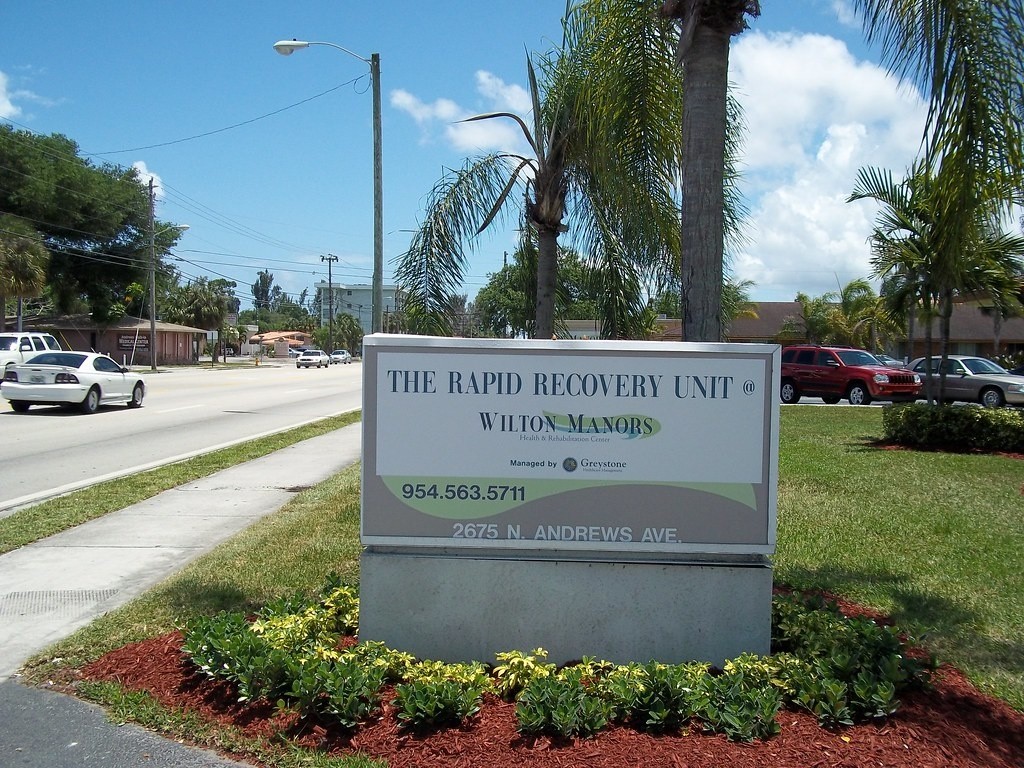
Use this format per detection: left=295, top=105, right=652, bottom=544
left=149, top=224, right=191, bottom=370
left=273, top=38, right=383, bottom=334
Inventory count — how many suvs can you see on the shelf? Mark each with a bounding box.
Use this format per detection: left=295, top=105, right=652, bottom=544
left=0, top=331, right=62, bottom=384
left=780, top=343, right=923, bottom=408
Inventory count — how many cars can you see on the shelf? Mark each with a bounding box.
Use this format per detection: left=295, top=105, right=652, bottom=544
left=330, top=349, right=351, bottom=364
left=861, top=354, right=904, bottom=370
left=0, top=350, right=147, bottom=414
left=289, top=347, right=308, bottom=359
left=222, top=348, right=235, bottom=356
left=900, top=352, right=1024, bottom=410
left=296, top=350, right=329, bottom=369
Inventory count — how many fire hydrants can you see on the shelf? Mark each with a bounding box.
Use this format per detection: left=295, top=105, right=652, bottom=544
left=255, top=357, right=260, bottom=366
